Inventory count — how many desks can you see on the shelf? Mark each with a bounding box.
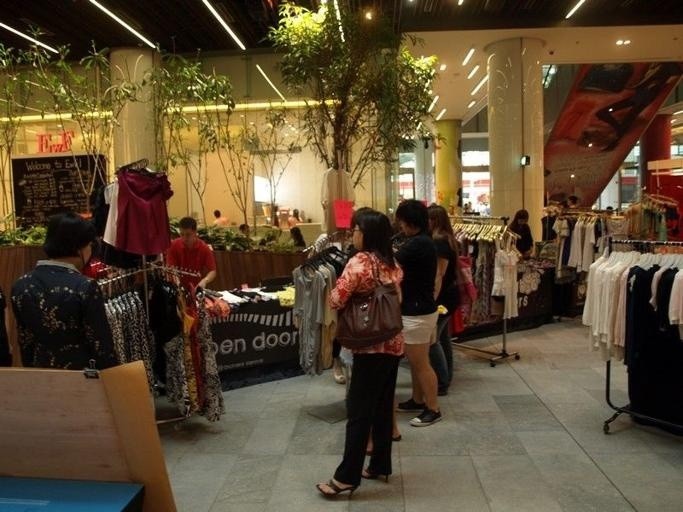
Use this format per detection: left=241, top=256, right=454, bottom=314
left=209, top=286, right=296, bottom=375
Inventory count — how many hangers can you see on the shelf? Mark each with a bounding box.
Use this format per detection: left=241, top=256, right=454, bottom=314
left=578, top=214, right=599, bottom=223
left=453, top=217, right=522, bottom=241
left=300, top=234, right=349, bottom=271
left=603, top=241, right=683, bottom=266
left=102, top=271, right=202, bottom=304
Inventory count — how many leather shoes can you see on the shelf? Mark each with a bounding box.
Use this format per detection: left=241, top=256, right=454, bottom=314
left=332, top=365, right=345, bottom=384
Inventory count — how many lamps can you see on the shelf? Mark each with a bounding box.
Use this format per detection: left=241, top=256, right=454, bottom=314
left=0, top=0, right=59, bottom=54
left=88, top=0, right=157, bottom=49
left=255, top=62, right=286, bottom=102
left=201, top=0, right=248, bottom=52
left=520, top=156, right=530, bottom=165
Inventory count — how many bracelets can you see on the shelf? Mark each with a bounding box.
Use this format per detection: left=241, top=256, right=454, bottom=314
left=205, top=277, right=208, bottom=283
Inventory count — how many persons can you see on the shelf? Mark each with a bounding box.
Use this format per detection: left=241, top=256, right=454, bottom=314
left=508, top=210, right=533, bottom=261
left=419, top=202, right=460, bottom=399
left=214, top=209, right=306, bottom=247
left=11, top=211, right=117, bottom=370
left=166, top=217, right=217, bottom=289
left=596, top=61, right=666, bottom=152
left=316, top=210, right=405, bottom=498
left=567, top=195, right=578, bottom=208
left=392, top=199, right=446, bottom=428
left=348, top=205, right=406, bottom=458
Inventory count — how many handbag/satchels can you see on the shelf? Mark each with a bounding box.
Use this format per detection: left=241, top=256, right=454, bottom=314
left=455, top=255, right=476, bottom=306
left=334, top=252, right=403, bottom=349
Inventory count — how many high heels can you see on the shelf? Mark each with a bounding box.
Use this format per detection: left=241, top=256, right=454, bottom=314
left=361, top=470, right=391, bottom=483
left=316, top=480, right=357, bottom=498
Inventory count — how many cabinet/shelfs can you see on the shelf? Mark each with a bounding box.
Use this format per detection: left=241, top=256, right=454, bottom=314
left=453, top=260, right=555, bottom=339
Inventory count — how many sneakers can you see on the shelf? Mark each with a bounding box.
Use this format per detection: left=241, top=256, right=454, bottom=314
left=394, top=399, right=425, bottom=413
left=408, top=407, right=441, bottom=426
